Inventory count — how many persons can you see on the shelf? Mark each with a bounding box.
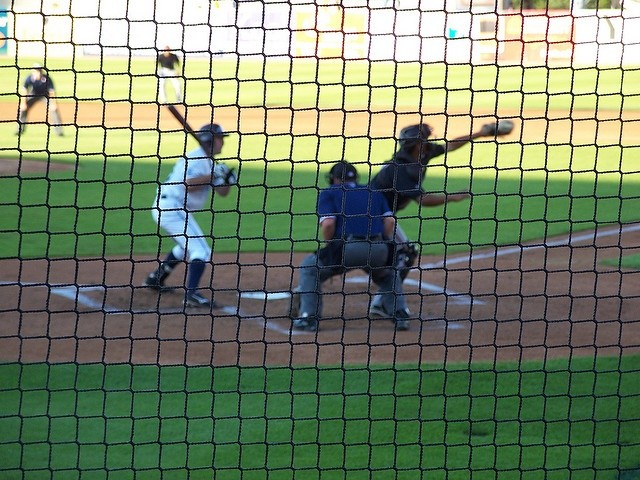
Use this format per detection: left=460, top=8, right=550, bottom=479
left=15, top=62, right=65, bottom=137
left=288, top=122, right=496, bottom=320
left=293, top=162, right=413, bottom=329
left=156, top=45, right=183, bottom=105
left=144, top=122, right=237, bottom=308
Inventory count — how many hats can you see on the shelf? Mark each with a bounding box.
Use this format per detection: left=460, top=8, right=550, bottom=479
left=325, top=163, right=356, bottom=187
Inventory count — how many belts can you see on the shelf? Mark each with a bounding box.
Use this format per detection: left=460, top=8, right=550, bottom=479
left=344, top=235, right=383, bottom=242
left=162, top=195, right=167, bottom=198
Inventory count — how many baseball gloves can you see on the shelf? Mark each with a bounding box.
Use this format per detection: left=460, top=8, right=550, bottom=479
left=482, top=120, right=513, bottom=135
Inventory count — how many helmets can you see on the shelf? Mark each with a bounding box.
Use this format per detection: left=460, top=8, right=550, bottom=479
left=196, top=123, right=229, bottom=149
left=399, top=124, right=436, bottom=159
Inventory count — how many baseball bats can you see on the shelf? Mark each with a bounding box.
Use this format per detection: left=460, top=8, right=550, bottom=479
left=165, top=105, right=219, bottom=165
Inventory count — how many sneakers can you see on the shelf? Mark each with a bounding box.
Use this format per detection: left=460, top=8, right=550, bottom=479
left=293, top=315, right=317, bottom=331
left=145, top=277, right=169, bottom=292
left=394, top=306, right=411, bottom=329
left=186, top=291, right=210, bottom=306
left=369, top=305, right=393, bottom=318
left=287, top=287, right=302, bottom=317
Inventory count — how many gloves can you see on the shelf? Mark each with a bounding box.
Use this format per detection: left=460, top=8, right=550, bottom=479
left=211, top=163, right=238, bottom=186
